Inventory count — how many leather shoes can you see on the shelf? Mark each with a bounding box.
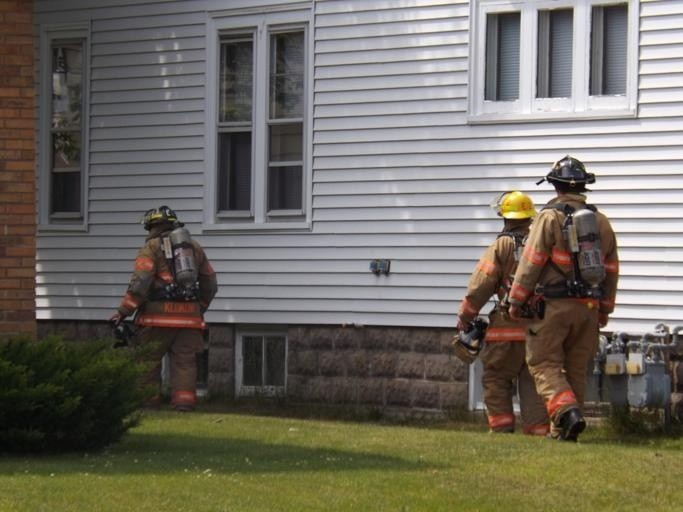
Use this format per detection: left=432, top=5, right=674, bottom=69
left=558, top=407, right=586, bottom=442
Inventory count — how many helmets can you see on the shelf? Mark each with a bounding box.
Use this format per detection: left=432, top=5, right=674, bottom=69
left=547, top=155, right=595, bottom=184
left=498, top=191, right=537, bottom=220
left=143, top=205, right=184, bottom=230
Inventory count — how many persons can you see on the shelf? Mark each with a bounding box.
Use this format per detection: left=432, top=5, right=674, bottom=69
left=456, top=190, right=551, bottom=436
left=508, top=155, right=619, bottom=443
left=110, top=206, right=217, bottom=412
